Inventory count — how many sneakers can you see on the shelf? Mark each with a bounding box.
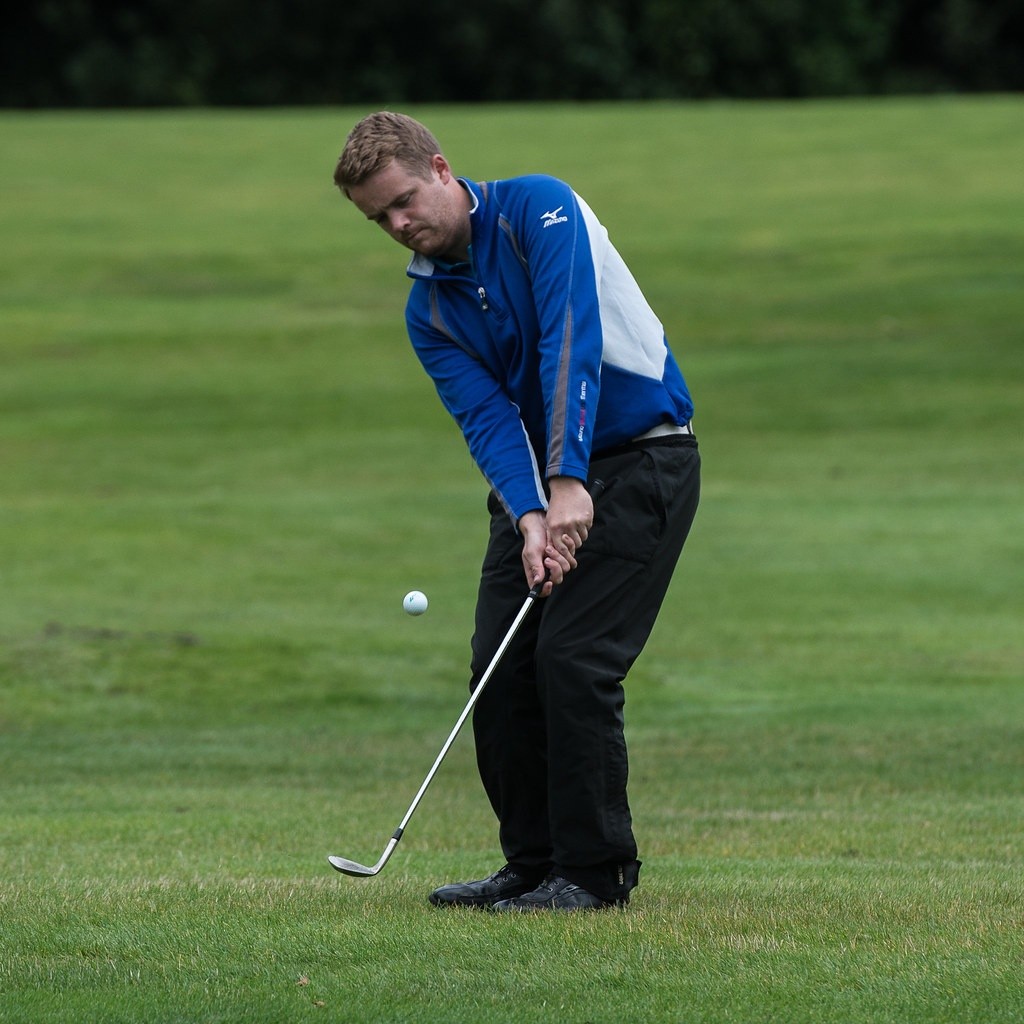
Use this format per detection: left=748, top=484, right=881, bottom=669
left=428, top=858, right=553, bottom=909
left=490, top=874, right=622, bottom=915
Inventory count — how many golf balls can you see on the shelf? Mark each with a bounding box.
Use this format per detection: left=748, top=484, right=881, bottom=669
left=402, top=591, right=430, bottom=615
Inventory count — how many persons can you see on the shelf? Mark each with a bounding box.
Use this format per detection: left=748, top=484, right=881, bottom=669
left=333, top=111, right=701, bottom=915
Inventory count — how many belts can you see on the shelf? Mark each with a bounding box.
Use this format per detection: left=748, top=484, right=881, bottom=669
left=633, top=419, right=694, bottom=441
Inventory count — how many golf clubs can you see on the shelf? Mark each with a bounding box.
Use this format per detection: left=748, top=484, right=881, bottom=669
left=326, top=480, right=609, bottom=879
